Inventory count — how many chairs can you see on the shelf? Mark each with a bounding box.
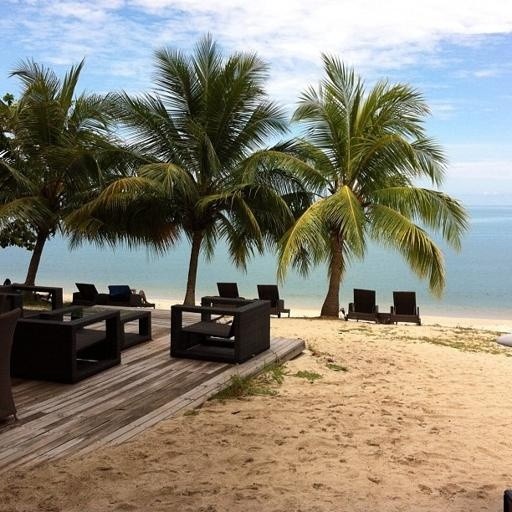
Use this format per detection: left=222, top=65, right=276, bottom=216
left=170, top=295, right=270, bottom=365
left=71, top=282, right=155, bottom=307
left=9, top=305, right=153, bottom=385
left=344, top=288, right=377, bottom=323
left=217, top=282, right=245, bottom=299
left=257, top=285, right=290, bottom=318
left=391, top=291, right=421, bottom=326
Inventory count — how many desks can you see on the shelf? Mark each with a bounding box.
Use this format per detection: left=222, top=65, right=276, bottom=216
left=58, top=306, right=152, bottom=349
